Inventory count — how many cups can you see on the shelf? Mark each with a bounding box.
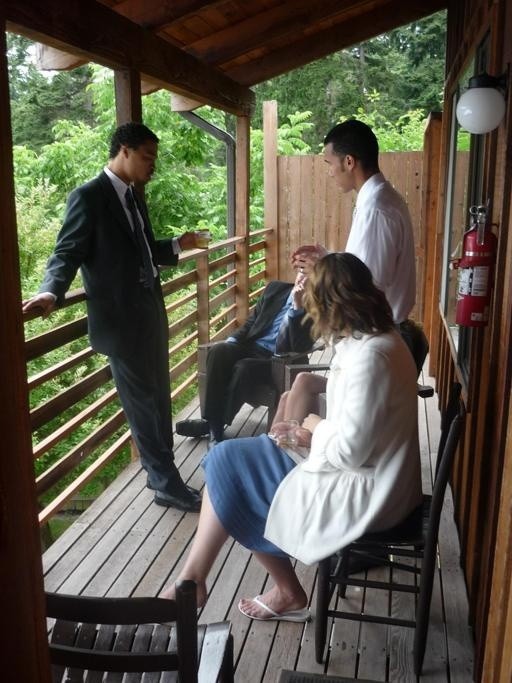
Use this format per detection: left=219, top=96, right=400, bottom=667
left=272, top=419, right=300, bottom=450
left=195, top=228, right=210, bottom=249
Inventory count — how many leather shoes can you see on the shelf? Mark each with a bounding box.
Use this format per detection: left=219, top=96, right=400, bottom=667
left=147, top=474, right=202, bottom=513
left=176, top=418, right=224, bottom=452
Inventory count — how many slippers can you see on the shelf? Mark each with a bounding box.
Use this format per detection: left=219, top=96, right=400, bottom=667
left=238, top=593, right=311, bottom=622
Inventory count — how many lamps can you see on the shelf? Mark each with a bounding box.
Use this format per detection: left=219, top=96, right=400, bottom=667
left=456, top=62, right=512, bottom=135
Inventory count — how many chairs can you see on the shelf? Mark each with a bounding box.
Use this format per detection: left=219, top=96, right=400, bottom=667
left=44, top=580, right=234, bottom=683
left=196, top=340, right=325, bottom=453
left=284, top=319, right=433, bottom=419
left=315, top=381, right=467, bottom=676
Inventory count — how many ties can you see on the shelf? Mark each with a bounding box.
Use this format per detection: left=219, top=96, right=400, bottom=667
left=125, top=189, right=154, bottom=290
left=258, top=299, right=291, bottom=352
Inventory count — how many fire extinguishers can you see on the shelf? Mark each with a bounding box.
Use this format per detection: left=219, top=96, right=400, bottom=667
left=455, top=205, right=496, bottom=328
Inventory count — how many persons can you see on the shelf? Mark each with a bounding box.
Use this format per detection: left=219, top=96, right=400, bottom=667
left=158, top=254, right=422, bottom=621
left=288, top=120, right=415, bottom=322
left=175, top=271, right=315, bottom=439
left=260, top=320, right=429, bottom=436
left=21, top=124, right=212, bottom=512
